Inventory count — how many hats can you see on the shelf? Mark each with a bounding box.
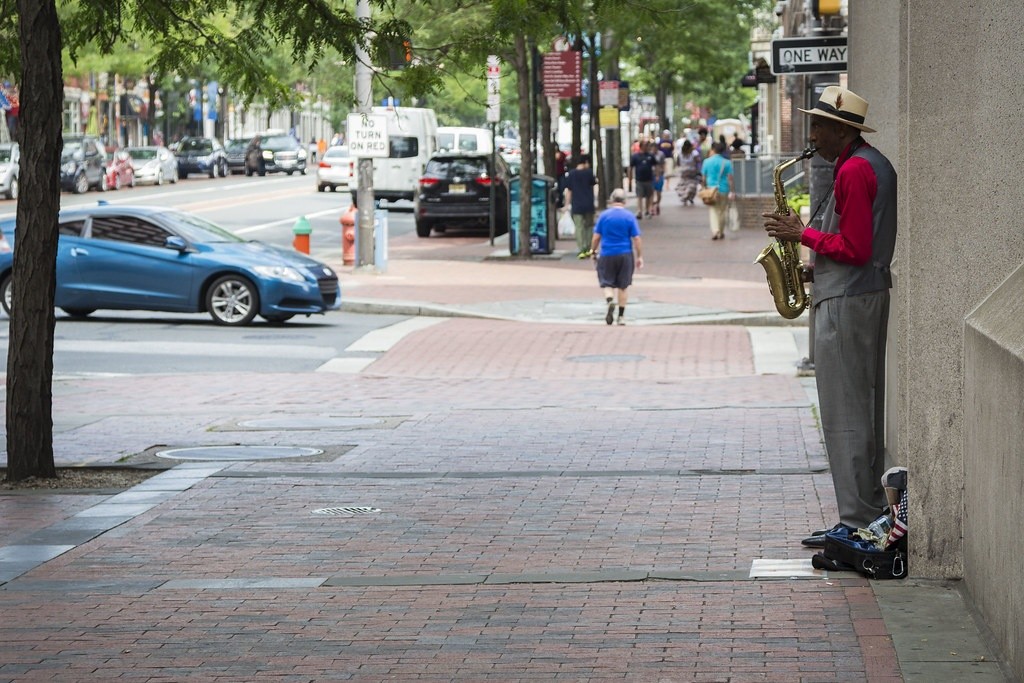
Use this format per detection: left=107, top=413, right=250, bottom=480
left=796, top=85, right=877, bottom=133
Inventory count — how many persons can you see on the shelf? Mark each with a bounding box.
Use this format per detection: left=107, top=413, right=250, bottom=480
left=589, top=187, right=644, bottom=325
left=330, top=132, right=343, bottom=145
left=309, top=136, right=316, bottom=164
left=702, top=140, right=736, bottom=242
left=552, top=127, right=746, bottom=224
left=564, top=152, right=595, bottom=261
left=762, top=85, right=898, bottom=552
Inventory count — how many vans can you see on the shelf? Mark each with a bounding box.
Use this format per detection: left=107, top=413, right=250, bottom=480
left=348, top=105, right=438, bottom=208
left=436, top=126, right=493, bottom=156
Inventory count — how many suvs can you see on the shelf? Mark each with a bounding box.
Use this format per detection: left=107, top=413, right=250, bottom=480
left=59, top=137, right=108, bottom=194
left=414, top=150, right=515, bottom=239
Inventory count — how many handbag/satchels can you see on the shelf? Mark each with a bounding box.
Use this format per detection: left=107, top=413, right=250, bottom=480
left=822, top=505, right=908, bottom=580
left=700, top=186, right=721, bottom=205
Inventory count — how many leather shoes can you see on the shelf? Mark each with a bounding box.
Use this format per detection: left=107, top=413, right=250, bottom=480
left=801, top=522, right=858, bottom=548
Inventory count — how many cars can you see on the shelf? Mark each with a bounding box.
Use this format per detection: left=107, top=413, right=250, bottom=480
left=495, top=134, right=537, bottom=177
left=0, top=201, right=343, bottom=327
left=316, top=145, right=350, bottom=191
left=102, top=145, right=179, bottom=190
left=174, top=131, right=308, bottom=180
left=0, top=141, right=21, bottom=199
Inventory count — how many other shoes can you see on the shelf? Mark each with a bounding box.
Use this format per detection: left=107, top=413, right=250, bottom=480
left=615, top=317, right=626, bottom=325
left=606, top=301, right=616, bottom=325
left=578, top=249, right=591, bottom=259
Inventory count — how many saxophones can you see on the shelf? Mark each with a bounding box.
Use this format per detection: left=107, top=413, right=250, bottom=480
left=751, top=147, right=817, bottom=321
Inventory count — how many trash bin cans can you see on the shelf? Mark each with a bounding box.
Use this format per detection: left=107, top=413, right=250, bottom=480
left=508, top=173, right=556, bottom=255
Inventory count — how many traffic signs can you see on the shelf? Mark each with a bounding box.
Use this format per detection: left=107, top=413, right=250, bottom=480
left=772, top=37, right=851, bottom=74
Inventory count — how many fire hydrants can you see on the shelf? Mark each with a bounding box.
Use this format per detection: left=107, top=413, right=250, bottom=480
left=339, top=210, right=357, bottom=265
left=292, top=215, right=312, bottom=256
